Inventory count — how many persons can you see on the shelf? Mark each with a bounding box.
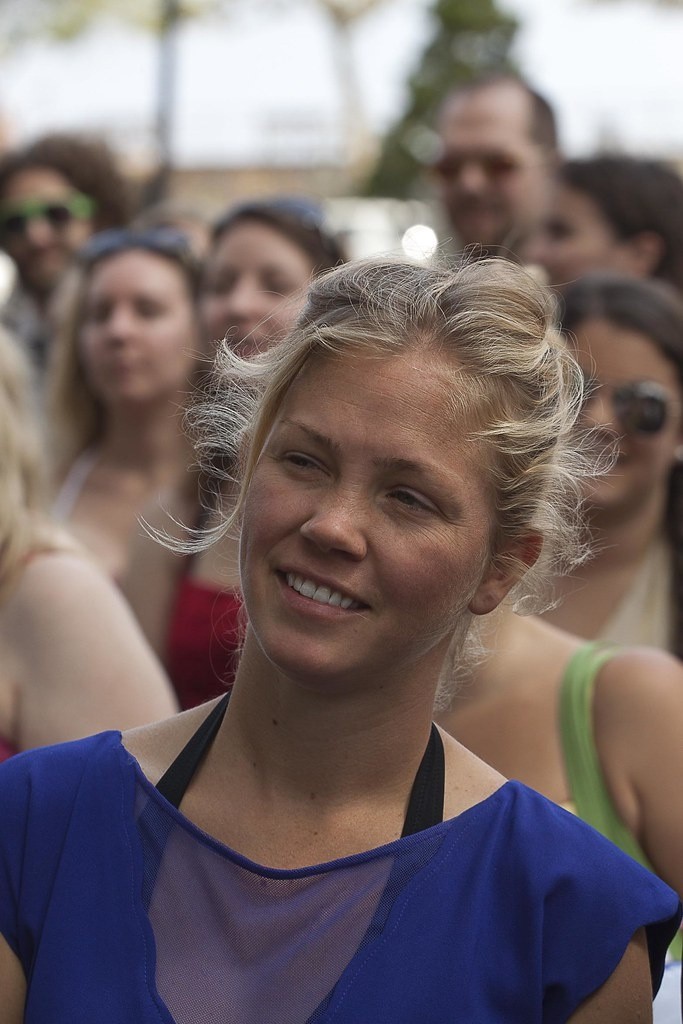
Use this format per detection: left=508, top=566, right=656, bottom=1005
left=1, top=256, right=682, bottom=1024
left=1, top=80, right=683, bottom=1024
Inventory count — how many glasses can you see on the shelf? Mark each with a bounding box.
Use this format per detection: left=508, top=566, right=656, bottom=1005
left=569, top=371, right=677, bottom=439
left=203, top=190, right=322, bottom=239
left=81, top=219, right=191, bottom=266
left=429, top=137, right=526, bottom=181
left=1, top=191, right=98, bottom=239
